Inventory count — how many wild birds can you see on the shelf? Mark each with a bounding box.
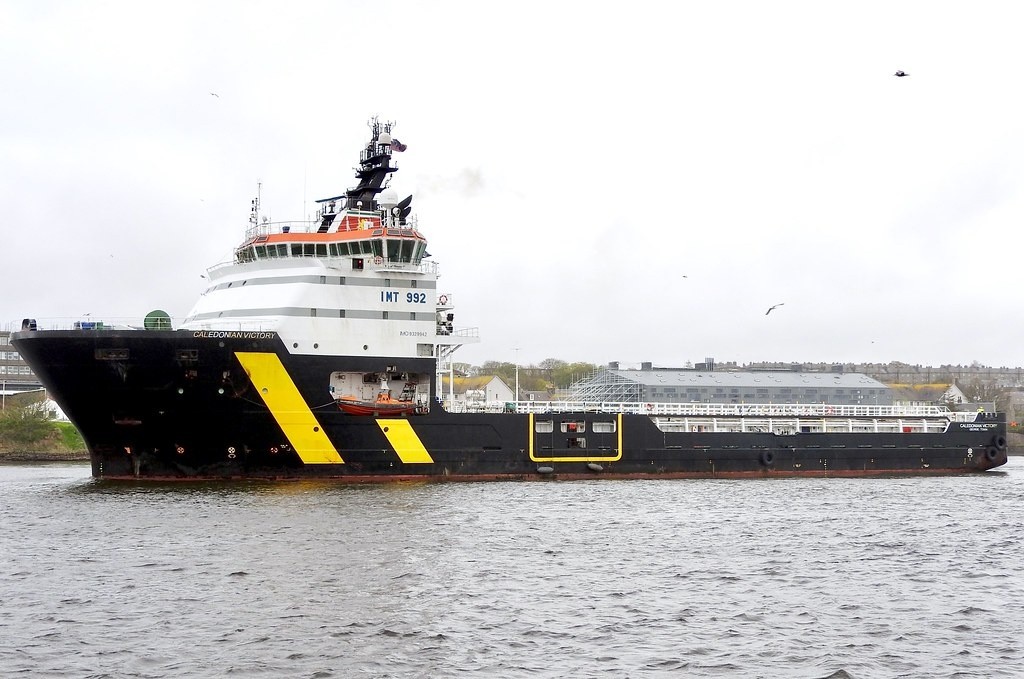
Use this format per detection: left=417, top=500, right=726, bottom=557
left=765, top=303, right=785, bottom=316
left=210, top=91, right=219, bottom=97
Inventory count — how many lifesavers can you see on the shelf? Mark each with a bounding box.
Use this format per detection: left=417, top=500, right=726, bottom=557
left=760, top=451, right=776, bottom=466
left=985, top=435, right=1007, bottom=462
left=439, top=294, right=448, bottom=305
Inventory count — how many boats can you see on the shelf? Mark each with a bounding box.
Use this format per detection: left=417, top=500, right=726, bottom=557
left=10, top=118, right=1010, bottom=484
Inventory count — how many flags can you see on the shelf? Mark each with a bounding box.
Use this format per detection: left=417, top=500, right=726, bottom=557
left=391, top=139, right=407, bottom=152
